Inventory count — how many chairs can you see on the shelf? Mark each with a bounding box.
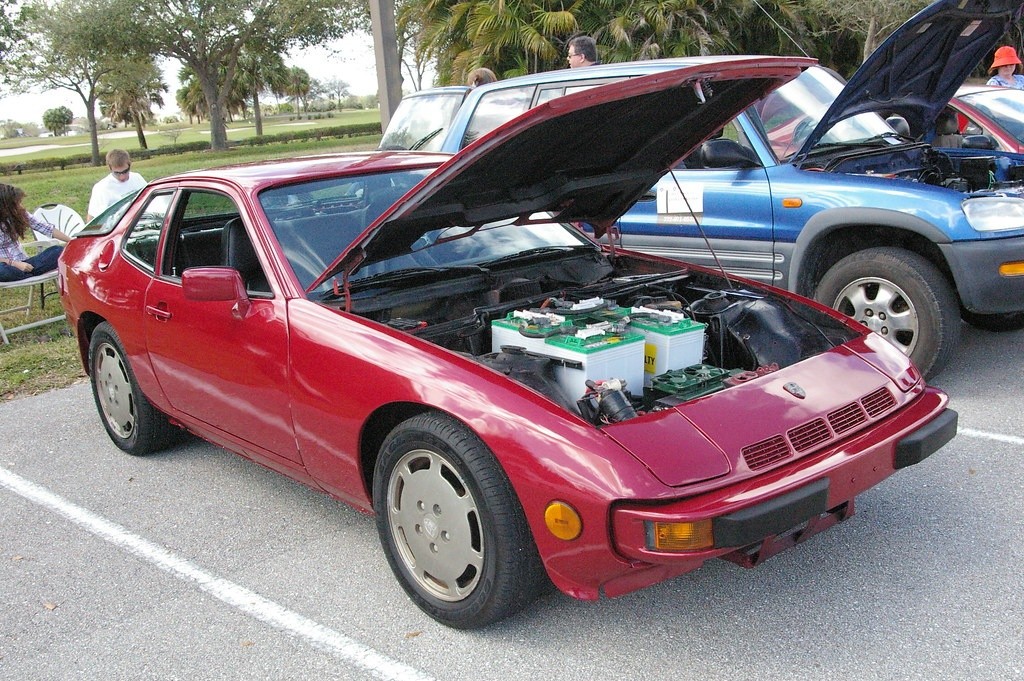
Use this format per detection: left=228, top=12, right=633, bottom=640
left=932, top=114, right=964, bottom=148
left=361, top=186, right=410, bottom=234
left=0, top=239, right=66, bottom=344
left=31, top=203, right=85, bottom=311
left=221, top=217, right=281, bottom=293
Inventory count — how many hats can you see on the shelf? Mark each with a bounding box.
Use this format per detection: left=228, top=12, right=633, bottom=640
left=988, top=46, right=1021, bottom=75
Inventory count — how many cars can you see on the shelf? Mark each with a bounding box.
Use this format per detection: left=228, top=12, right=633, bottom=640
left=58, top=56, right=959, bottom=630
left=439, top=0, right=1023, bottom=382
left=339, top=86, right=477, bottom=198
left=765, top=85, right=1024, bottom=172
left=39, top=125, right=85, bottom=138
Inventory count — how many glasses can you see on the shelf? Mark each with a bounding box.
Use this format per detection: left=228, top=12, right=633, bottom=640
left=114, top=165, right=130, bottom=174
left=569, top=53, right=578, bottom=58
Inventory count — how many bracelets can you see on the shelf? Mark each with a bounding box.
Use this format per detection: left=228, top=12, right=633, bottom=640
left=8, top=259, right=14, bottom=265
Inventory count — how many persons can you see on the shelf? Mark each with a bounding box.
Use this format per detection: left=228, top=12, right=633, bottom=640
left=87, top=149, right=148, bottom=226
left=567, top=36, right=602, bottom=67
left=986, top=46, right=1024, bottom=90
left=0, top=183, right=70, bottom=283
left=468, top=67, right=497, bottom=89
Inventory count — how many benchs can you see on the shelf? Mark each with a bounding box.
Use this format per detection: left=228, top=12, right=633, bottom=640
left=127, top=210, right=361, bottom=277
